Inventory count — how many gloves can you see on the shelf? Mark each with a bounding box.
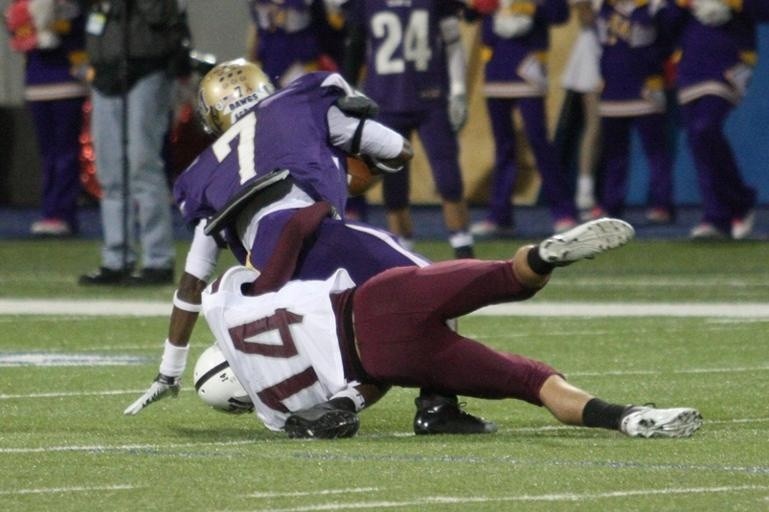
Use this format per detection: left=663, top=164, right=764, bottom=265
left=124, top=376, right=181, bottom=416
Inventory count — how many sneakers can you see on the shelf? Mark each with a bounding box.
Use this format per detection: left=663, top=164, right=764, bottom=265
left=288, top=402, right=362, bottom=439
left=539, top=216, right=638, bottom=264
left=122, top=266, right=175, bottom=285
left=730, top=206, right=757, bottom=238
left=619, top=402, right=704, bottom=440
left=80, top=262, right=134, bottom=284
left=413, top=395, right=497, bottom=433
left=689, top=222, right=723, bottom=237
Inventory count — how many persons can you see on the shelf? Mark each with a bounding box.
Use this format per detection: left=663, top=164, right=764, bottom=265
left=244, top=0, right=769, bottom=243
left=193, top=201, right=704, bottom=439
left=1, top=0, right=215, bottom=287
left=124, top=59, right=496, bottom=439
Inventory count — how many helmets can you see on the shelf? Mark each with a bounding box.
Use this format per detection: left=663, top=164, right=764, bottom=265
left=193, top=341, right=257, bottom=416
left=195, top=58, right=274, bottom=137
